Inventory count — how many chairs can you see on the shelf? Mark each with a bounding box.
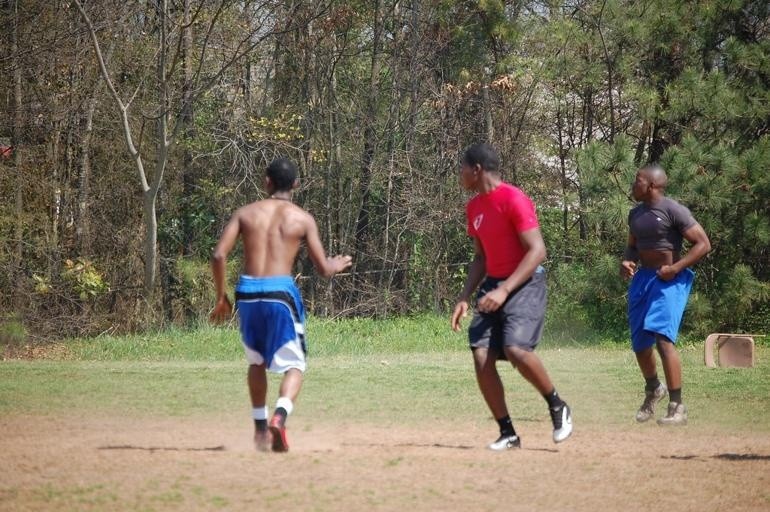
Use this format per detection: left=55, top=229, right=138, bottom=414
left=703, top=332, right=766, bottom=370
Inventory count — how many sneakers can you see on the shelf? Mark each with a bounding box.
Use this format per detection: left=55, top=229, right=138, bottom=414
left=255, top=408, right=290, bottom=452
left=656, top=401, right=688, bottom=424
left=550, top=402, right=572, bottom=442
left=487, top=433, right=521, bottom=449
left=636, top=383, right=667, bottom=421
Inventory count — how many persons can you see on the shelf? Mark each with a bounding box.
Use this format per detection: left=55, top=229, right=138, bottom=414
left=620, top=165, right=711, bottom=426
left=451, top=145, right=573, bottom=451
left=208, top=159, right=353, bottom=452
